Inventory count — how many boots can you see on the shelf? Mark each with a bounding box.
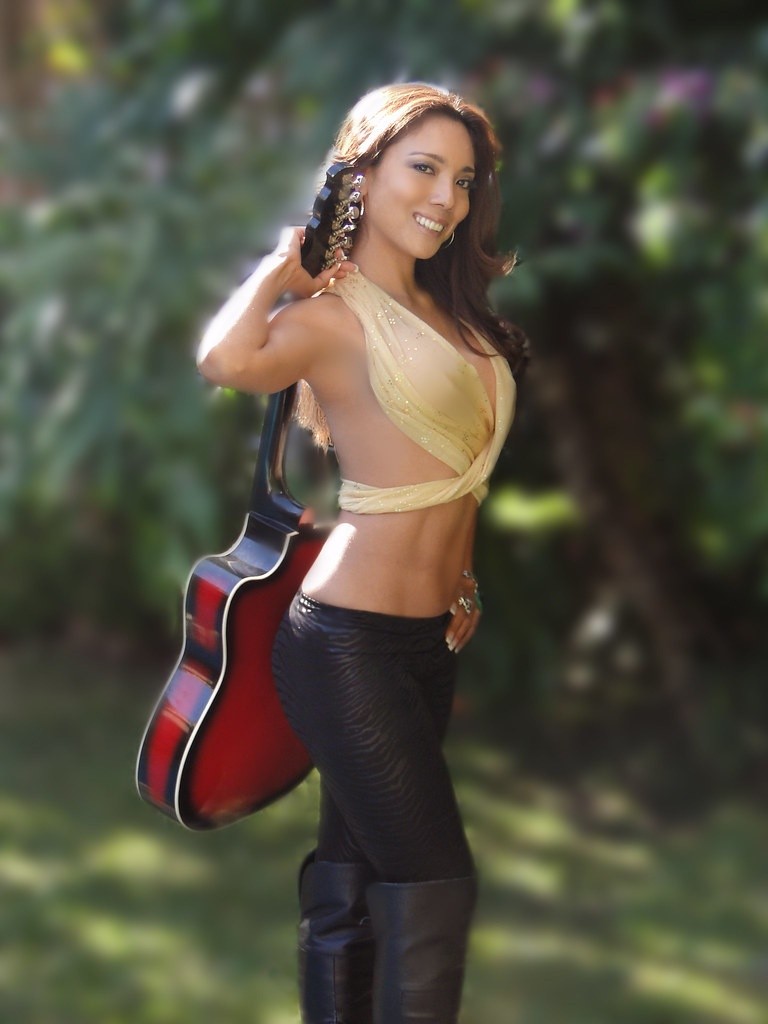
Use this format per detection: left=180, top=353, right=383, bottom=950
left=364, top=871, right=477, bottom=1024
left=298, top=849, right=376, bottom=1024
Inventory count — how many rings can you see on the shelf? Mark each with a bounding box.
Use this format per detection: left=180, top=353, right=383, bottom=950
left=457, top=596, right=472, bottom=615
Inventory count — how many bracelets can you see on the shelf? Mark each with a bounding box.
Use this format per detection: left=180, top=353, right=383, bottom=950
left=461, top=569, right=478, bottom=595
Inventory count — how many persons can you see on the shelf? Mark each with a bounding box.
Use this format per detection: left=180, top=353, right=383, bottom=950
left=196, top=81, right=529, bottom=1024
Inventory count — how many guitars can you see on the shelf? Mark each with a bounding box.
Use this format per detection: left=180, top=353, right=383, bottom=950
left=132, top=148, right=373, bottom=839
